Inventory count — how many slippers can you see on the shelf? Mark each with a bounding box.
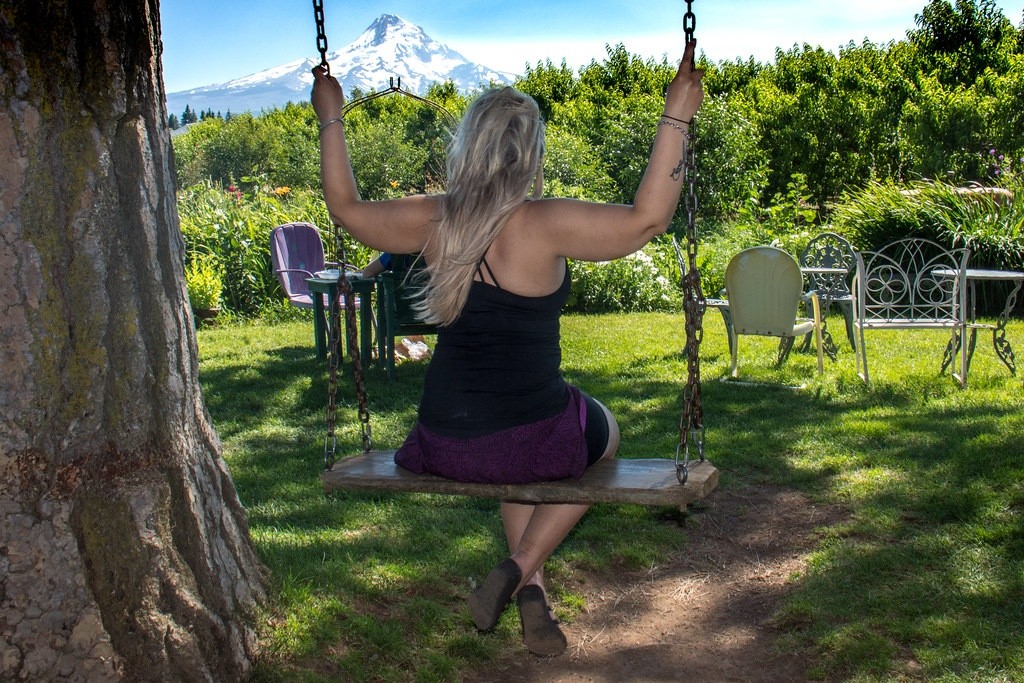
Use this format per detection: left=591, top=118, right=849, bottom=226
left=517, top=584, right=568, bottom=657
left=468, top=557, right=522, bottom=630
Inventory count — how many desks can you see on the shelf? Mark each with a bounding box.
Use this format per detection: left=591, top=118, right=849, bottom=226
left=306, top=276, right=374, bottom=364
left=800, top=266, right=850, bottom=361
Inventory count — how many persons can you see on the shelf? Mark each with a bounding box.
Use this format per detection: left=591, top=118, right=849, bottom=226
left=309, top=39, right=703, bottom=656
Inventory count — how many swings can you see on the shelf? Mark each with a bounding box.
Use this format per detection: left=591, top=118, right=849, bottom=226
left=313, top=0, right=722, bottom=504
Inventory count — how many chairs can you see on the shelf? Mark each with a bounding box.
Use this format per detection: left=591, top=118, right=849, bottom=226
left=801, top=230, right=856, bottom=354
left=673, top=236, right=730, bottom=362
left=850, top=236, right=974, bottom=392
left=718, top=244, right=829, bottom=393
left=930, top=266, right=1024, bottom=381
left=269, top=218, right=379, bottom=351
left=373, top=268, right=440, bottom=377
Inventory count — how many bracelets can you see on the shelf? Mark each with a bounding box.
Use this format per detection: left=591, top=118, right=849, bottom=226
left=319, top=119, right=345, bottom=134
left=658, top=114, right=692, bottom=138
